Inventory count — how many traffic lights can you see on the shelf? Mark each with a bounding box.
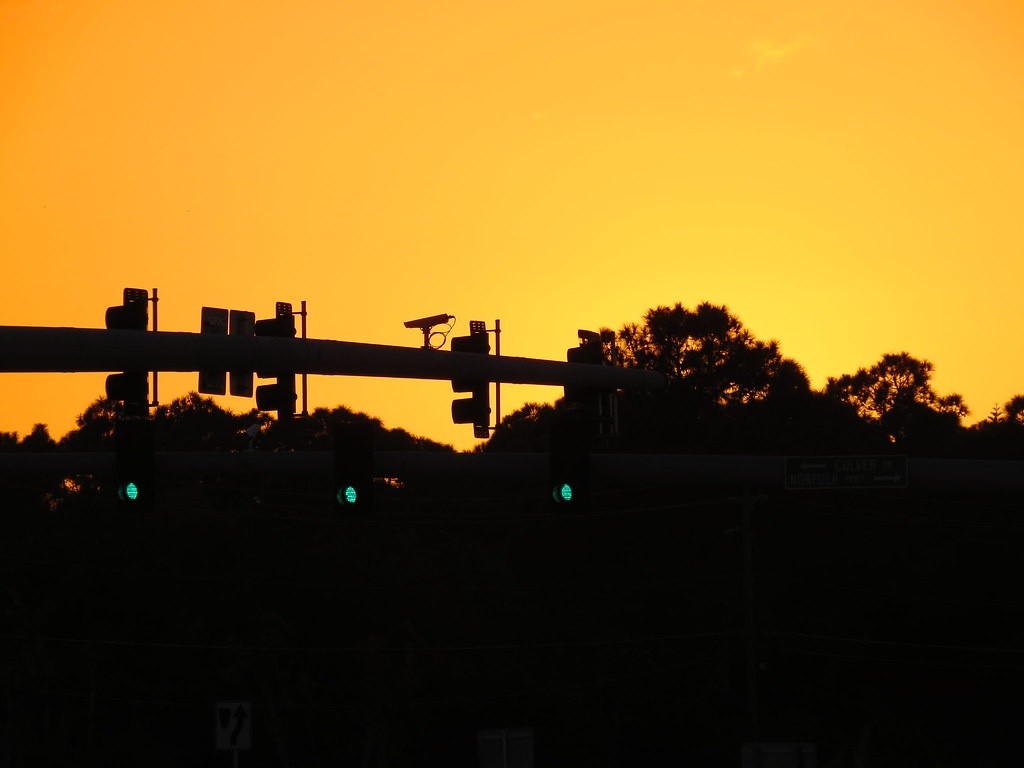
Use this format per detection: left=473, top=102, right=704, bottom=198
left=450, top=332, right=490, bottom=427
left=112, top=419, right=150, bottom=506
left=252, top=315, right=295, bottom=412
left=331, top=425, right=373, bottom=513
left=544, top=429, right=586, bottom=507
left=105, top=302, right=147, bottom=415
